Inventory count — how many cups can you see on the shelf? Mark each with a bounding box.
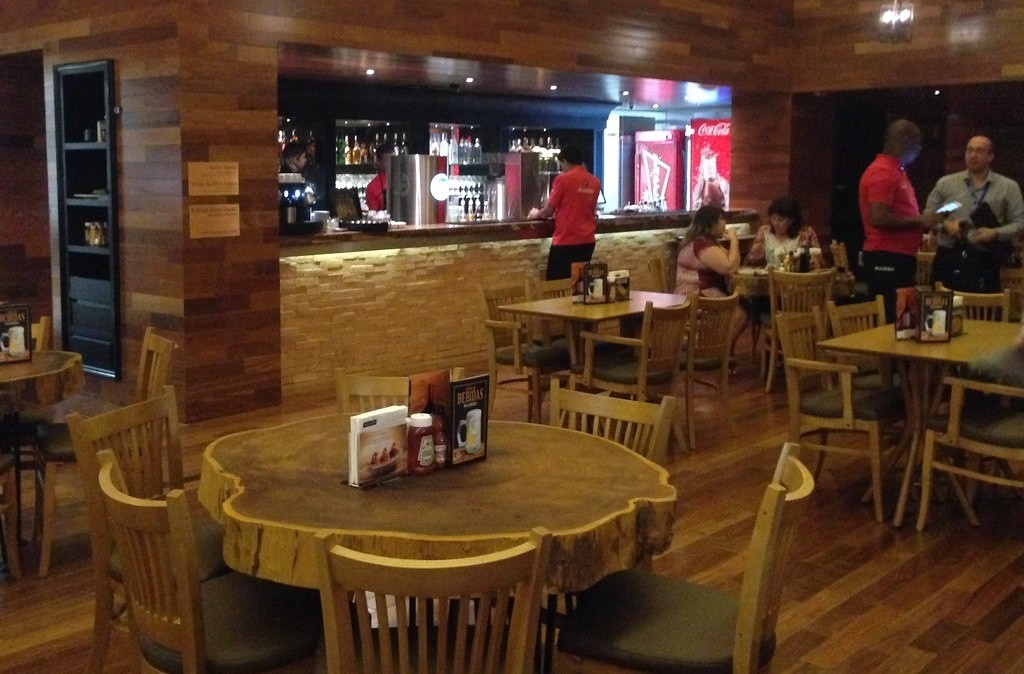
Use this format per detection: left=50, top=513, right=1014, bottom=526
left=924, top=310, right=947, bottom=337
left=456, top=408, right=482, bottom=455
left=588, top=278, right=603, bottom=300
left=0, top=326, right=25, bottom=357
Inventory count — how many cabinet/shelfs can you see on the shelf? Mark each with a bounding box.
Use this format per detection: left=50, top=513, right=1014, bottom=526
left=52, top=57, right=122, bottom=380
left=278, top=77, right=622, bottom=218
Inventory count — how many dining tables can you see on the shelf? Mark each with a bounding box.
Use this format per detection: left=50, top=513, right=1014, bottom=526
left=727, top=253, right=841, bottom=362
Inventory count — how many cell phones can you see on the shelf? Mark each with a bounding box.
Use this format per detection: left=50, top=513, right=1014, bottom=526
left=936, top=201, right=963, bottom=213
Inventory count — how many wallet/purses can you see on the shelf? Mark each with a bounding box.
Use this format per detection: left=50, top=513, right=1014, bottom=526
left=969, top=201, right=1015, bottom=260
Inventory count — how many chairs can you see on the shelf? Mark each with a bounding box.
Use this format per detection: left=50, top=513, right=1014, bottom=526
left=0, top=229, right=1024, bottom=674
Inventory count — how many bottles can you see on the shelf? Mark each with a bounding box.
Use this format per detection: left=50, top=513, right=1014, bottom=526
left=84, top=221, right=109, bottom=248
left=695, top=151, right=727, bottom=210
left=903, top=296, right=911, bottom=328
left=405, top=412, right=450, bottom=477
left=428, top=132, right=506, bottom=225
left=275, top=127, right=408, bottom=235
left=639, top=198, right=668, bottom=213
left=509, top=136, right=564, bottom=173
left=768, top=244, right=824, bottom=272
left=423, top=385, right=437, bottom=416
left=577, top=268, right=584, bottom=294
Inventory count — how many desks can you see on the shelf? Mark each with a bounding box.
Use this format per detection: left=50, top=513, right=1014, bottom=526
left=817, top=307, right=1024, bottom=526
left=196, top=408, right=679, bottom=602
left=496, top=285, right=695, bottom=367
left=0, top=348, right=86, bottom=548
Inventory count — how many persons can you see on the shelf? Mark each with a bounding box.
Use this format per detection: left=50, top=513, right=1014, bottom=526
left=526, top=144, right=600, bottom=346
left=368, top=442, right=400, bottom=466
left=742, top=198, right=821, bottom=319
left=859, top=119, right=956, bottom=420
left=366, top=144, right=396, bottom=211
left=926, top=135, right=1024, bottom=415
left=672, top=205, right=748, bottom=377
left=279, top=142, right=310, bottom=222
